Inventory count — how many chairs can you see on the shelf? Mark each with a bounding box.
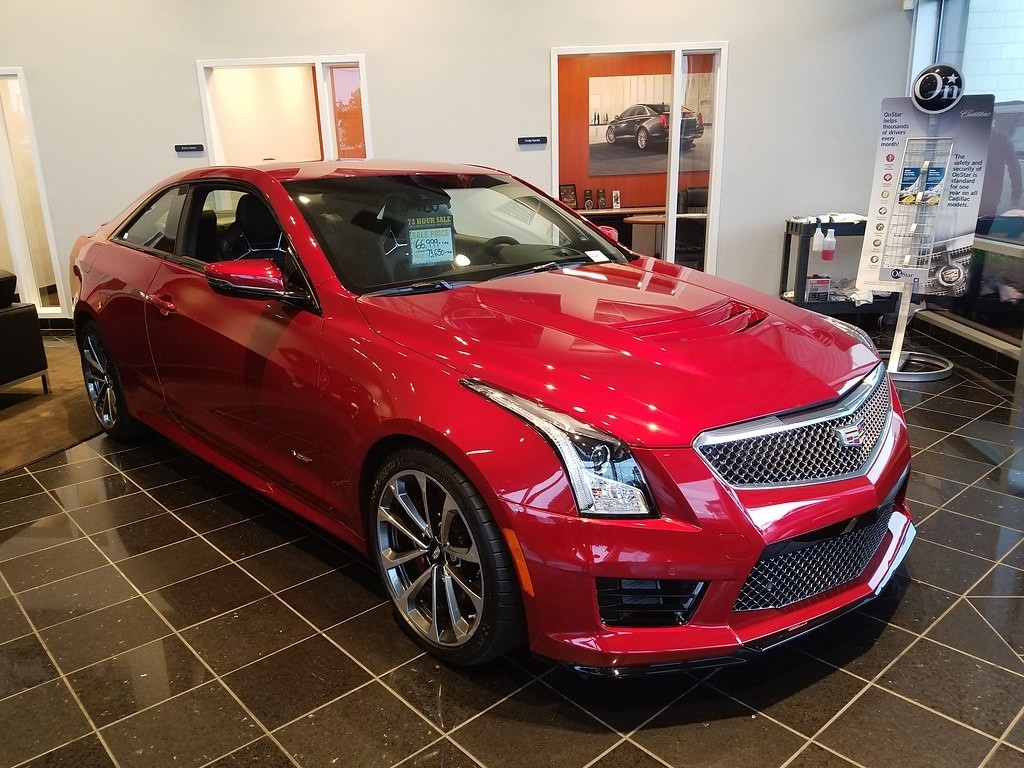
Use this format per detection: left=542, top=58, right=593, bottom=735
left=317, top=212, right=349, bottom=253
left=0, top=268, right=54, bottom=410
left=165, top=210, right=224, bottom=264
left=677, top=186, right=708, bottom=272
left=365, top=192, right=456, bottom=285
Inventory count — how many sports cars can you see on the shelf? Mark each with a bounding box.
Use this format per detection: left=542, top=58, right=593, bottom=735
left=66, top=156, right=920, bottom=680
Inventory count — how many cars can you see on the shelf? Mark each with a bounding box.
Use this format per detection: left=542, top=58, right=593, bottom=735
left=604, top=101, right=705, bottom=151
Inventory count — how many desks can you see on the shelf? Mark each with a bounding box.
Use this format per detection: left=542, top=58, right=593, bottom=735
left=575, top=206, right=666, bottom=259
left=623, top=214, right=665, bottom=261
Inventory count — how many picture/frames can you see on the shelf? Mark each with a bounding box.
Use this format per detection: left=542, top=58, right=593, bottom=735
left=558, top=184, right=579, bottom=210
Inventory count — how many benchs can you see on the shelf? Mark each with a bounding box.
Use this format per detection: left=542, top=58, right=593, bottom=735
left=219, top=193, right=291, bottom=269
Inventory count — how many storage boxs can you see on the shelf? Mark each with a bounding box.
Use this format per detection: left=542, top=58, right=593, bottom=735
left=803, top=276, right=831, bottom=302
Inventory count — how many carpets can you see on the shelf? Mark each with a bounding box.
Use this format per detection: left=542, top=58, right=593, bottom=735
left=0, top=345, right=107, bottom=475
